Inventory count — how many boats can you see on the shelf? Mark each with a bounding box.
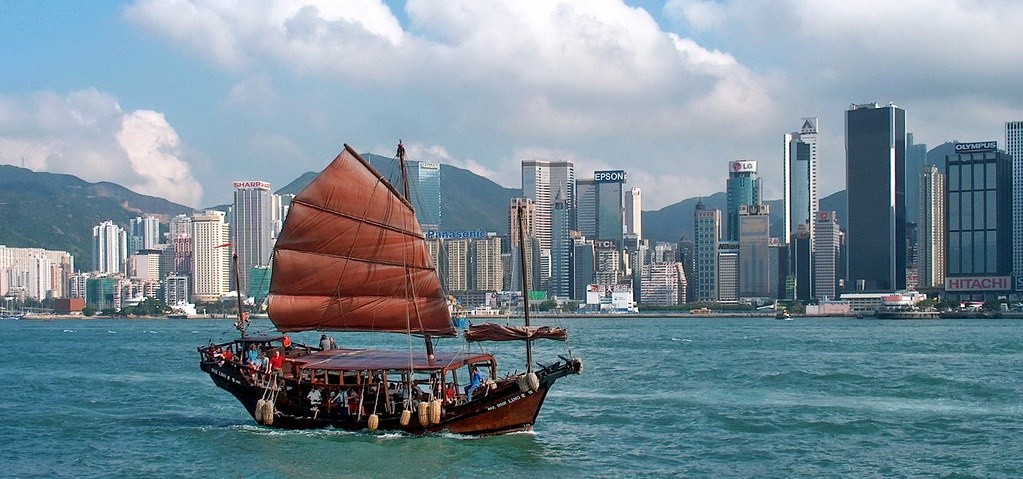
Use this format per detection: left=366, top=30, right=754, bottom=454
left=774, top=310, right=791, bottom=321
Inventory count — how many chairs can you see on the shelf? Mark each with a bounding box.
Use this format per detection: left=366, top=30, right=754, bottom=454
left=283, top=362, right=293, bottom=379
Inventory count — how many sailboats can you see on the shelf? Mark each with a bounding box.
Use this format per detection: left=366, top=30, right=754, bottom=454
left=190, top=136, right=586, bottom=437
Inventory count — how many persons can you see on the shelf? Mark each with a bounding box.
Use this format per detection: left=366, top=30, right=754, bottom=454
left=388, top=383, right=423, bottom=400
left=464, top=367, right=486, bottom=402
left=370, top=378, right=382, bottom=395
left=220, top=345, right=234, bottom=361
left=283, top=336, right=291, bottom=350
left=332, top=384, right=358, bottom=405
left=307, top=385, right=327, bottom=403
left=268, top=350, right=296, bottom=376
left=445, top=382, right=457, bottom=403
left=213, top=347, right=223, bottom=367
left=330, top=337, right=337, bottom=349
left=245, top=343, right=269, bottom=386
left=206, top=343, right=215, bottom=352
left=319, top=335, right=326, bottom=351
